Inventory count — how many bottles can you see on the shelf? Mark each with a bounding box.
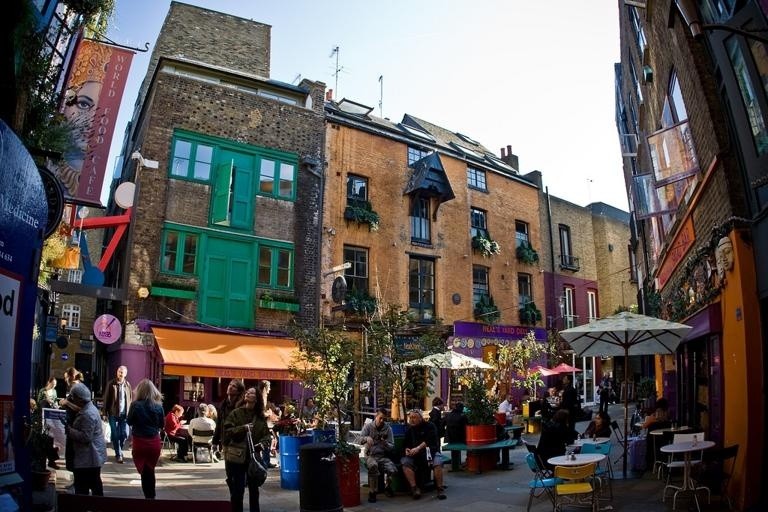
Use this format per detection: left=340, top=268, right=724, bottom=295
left=570, top=451, right=576, bottom=461
left=577, top=434, right=581, bottom=443
left=693, top=435, right=697, bottom=446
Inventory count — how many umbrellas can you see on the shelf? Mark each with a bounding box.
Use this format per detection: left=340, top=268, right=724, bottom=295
left=551, top=363, right=581, bottom=372
left=517, top=366, right=559, bottom=400
left=403, top=349, right=493, bottom=410
left=558, top=310, right=692, bottom=475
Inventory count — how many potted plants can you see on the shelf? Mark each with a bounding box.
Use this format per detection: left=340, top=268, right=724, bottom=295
left=278, top=303, right=542, bottom=511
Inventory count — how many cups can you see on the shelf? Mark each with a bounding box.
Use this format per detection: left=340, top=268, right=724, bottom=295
left=671, top=421, right=678, bottom=429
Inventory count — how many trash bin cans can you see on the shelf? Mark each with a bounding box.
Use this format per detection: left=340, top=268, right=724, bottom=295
left=299, top=441, right=344, bottom=512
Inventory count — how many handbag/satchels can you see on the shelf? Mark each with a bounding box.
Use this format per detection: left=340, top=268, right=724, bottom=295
left=224, top=446, right=267, bottom=482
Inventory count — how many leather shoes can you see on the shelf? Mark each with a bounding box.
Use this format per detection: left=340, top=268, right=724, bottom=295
left=117, top=456, right=123, bottom=462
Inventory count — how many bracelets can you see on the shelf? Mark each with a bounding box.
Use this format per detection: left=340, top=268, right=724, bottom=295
left=416, top=446, right=420, bottom=450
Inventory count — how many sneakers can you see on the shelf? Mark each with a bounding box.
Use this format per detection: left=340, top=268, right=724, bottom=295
left=368, top=486, right=393, bottom=503
left=437, top=487, right=446, bottom=499
left=412, top=487, right=420, bottom=500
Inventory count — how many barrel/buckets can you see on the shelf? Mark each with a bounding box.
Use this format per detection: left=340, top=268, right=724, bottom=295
left=335, top=452, right=361, bottom=507
left=312, top=424, right=336, bottom=443
left=391, top=424, right=407, bottom=437
left=465, top=424, right=497, bottom=475
left=279, top=435, right=314, bottom=491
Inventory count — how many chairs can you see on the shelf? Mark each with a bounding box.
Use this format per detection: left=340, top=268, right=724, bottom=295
left=155, top=415, right=279, bottom=467
left=521, top=414, right=741, bottom=511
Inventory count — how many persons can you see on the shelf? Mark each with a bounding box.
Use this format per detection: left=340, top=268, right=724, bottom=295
left=172, top=378, right=318, bottom=512
left=46, top=80, right=104, bottom=204
left=428, top=397, right=446, bottom=436
left=359, top=407, right=398, bottom=503
left=497, top=371, right=616, bottom=470
left=126, top=379, right=163, bottom=501
left=630, top=397, right=671, bottom=478
left=400, top=408, right=445, bottom=499
left=30, top=366, right=108, bottom=498
left=445, top=401, right=466, bottom=445
left=716, top=237, right=733, bottom=269
left=104, top=366, right=132, bottom=462
